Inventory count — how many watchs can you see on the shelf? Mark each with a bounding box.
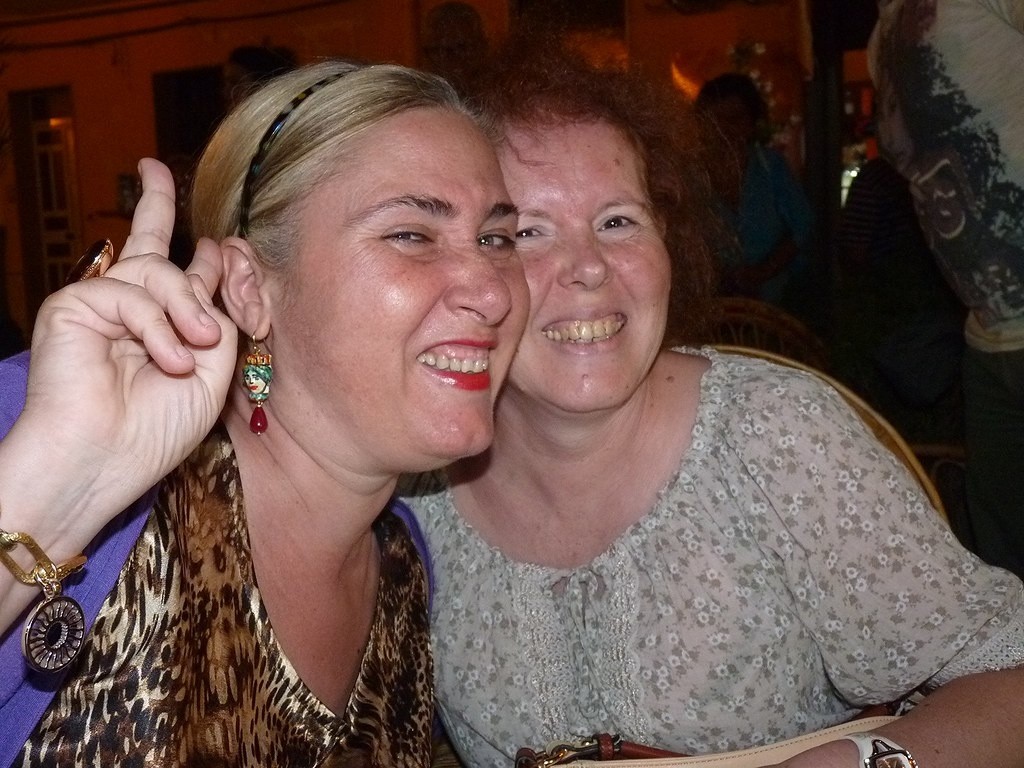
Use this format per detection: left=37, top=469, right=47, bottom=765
left=839, top=731, right=919, bottom=768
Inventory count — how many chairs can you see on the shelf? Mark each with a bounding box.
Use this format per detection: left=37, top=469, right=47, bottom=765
left=661, top=299, right=985, bottom=563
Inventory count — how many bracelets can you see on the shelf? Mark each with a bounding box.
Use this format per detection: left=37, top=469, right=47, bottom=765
left=0, top=526, right=90, bottom=680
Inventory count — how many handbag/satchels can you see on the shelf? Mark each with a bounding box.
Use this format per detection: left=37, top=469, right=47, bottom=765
left=513, top=701, right=903, bottom=768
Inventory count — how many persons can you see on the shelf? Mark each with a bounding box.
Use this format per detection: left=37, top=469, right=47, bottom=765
left=693, top=0, right=1023, bottom=578
left=393, top=46, right=1024, bottom=768
left=0, top=61, right=532, bottom=768
left=221, top=41, right=298, bottom=110
